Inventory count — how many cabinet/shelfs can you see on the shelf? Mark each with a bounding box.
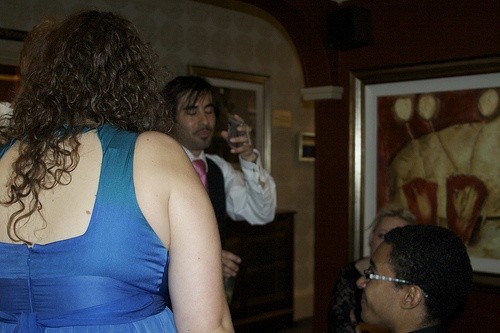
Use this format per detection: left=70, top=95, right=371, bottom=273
left=223, top=209, right=299, bottom=333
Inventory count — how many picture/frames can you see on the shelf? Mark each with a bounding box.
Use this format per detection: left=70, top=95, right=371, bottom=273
left=297, top=131, right=316, bottom=163
left=347, top=55, right=500, bottom=292
left=0, top=26, right=32, bottom=150
left=185, top=63, right=272, bottom=176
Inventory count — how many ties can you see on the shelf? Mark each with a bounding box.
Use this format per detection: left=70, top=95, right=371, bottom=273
left=190, top=159, right=209, bottom=195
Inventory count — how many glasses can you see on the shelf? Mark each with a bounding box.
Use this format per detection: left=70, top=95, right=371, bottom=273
left=363, top=269, right=429, bottom=298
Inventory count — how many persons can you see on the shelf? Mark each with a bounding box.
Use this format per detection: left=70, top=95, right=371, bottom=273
left=0, top=11, right=234, bottom=333
left=329, top=204, right=416, bottom=333
left=163, top=74, right=277, bottom=278
left=357, top=224, right=473, bottom=333
